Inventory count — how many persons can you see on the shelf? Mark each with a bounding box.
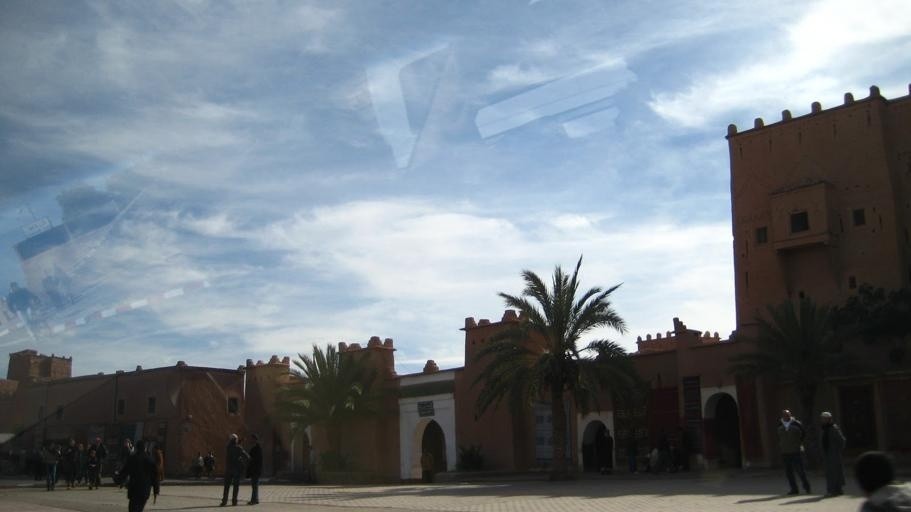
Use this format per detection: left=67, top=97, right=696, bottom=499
left=420, top=442, right=434, bottom=482
left=778, top=408, right=811, bottom=495
left=819, top=411, right=847, bottom=498
left=596, top=422, right=690, bottom=478
left=305, top=444, right=317, bottom=481
left=854, top=449, right=910, bottom=511
left=0, top=432, right=264, bottom=510
